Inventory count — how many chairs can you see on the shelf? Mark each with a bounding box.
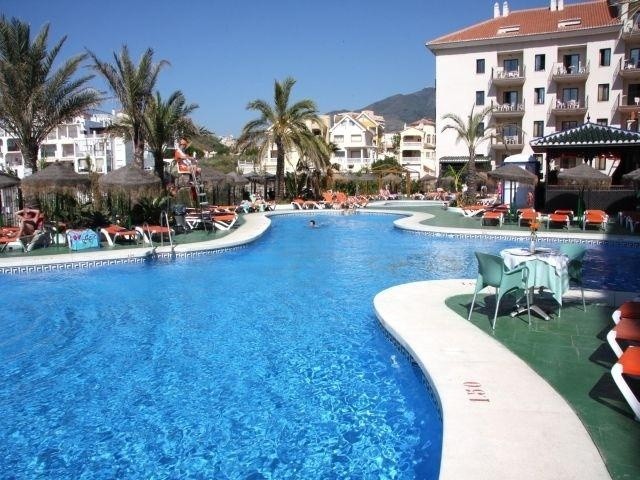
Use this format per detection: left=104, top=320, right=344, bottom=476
left=558, top=244, right=587, bottom=317
left=100, top=205, right=239, bottom=246
left=0, top=212, right=45, bottom=254
left=481, top=206, right=640, bottom=233
left=607, top=299, right=640, bottom=418
left=291, top=194, right=388, bottom=209
left=468, top=251, right=532, bottom=329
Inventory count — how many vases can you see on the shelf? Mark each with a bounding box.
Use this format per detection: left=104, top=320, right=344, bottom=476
left=530, top=241, right=535, bottom=252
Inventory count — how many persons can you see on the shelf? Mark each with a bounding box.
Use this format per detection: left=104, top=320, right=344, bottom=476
left=306, top=219, right=321, bottom=229
left=3, top=207, right=39, bottom=240
left=174, top=138, right=202, bottom=181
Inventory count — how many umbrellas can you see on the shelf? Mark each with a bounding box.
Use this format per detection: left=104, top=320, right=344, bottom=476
left=204, top=171, right=279, bottom=205
left=0, top=171, right=21, bottom=191
left=488, top=160, right=537, bottom=210
left=336, top=170, right=439, bottom=202
left=620, top=168, right=640, bottom=190
left=21, top=159, right=91, bottom=249
left=558, top=163, right=612, bottom=223
left=98, top=162, right=162, bottom=245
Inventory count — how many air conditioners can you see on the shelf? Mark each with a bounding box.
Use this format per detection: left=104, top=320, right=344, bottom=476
left=503, top=135, right=518, bottom=144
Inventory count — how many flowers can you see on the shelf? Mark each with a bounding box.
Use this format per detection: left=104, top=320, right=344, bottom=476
left=529, top=222, right=540, bottom=240
